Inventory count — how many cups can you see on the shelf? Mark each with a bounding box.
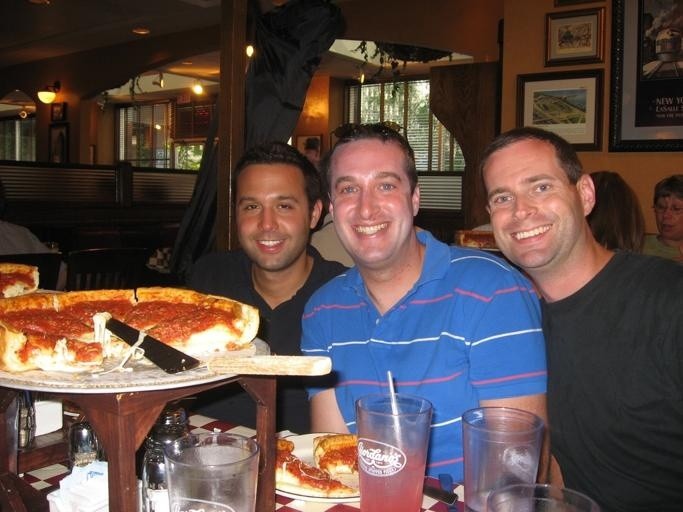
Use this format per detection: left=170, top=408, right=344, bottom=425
left=461, top=406, right=550, bottom=511
left=162, top=431, right=260, bottom=512
left=354, top=393, right=432, bottom=512
left=488, top=479, right=601, bottom=511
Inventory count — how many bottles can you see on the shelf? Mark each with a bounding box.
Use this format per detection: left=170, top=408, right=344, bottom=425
left=158, top=404, right=193, bottom=454
left=17, top=405, right=34, bottom=449
left=69, top=421, right=107, bottom=465
left=142, top=424, right=197, bottom=511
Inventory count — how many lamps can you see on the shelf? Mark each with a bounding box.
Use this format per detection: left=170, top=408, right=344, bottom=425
left=36, top=77, right=62, bottom=104
left=189, top=73, right=206, bottom=95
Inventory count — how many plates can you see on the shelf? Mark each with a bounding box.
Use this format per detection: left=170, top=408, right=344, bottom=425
left=275, top=430, right=372, bottom=504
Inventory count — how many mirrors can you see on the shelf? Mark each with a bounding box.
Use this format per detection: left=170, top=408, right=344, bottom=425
left=1, top=1, right=224, bottom=173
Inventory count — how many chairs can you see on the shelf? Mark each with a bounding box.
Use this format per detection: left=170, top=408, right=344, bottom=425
left=0, top=252, right=63, bottom=291
left=62, top=244, right=151, bottom=289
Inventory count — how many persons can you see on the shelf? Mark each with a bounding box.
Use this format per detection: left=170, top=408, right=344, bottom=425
left=469, top=116, right=683, bottom=512
left=173, top=139, right=351, bottom=432
left=585, top=165, right=646, bottom=255
left=301, top=123, right=554, bottom=498
left=635, top=172, right=683, bottom=262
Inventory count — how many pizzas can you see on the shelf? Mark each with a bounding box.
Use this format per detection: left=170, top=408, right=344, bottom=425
left=275, top=439, right=360, bottom=498
left=313, top=434, right=359, bottom=475
left=0, top=263, right=39, bottom=298
left=0, top=286, right=260, bottom=373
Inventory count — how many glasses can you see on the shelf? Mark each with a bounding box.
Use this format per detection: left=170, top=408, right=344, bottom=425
left=653, top=203, right=682, bottom=215
left=329, top=120, right=408, bottom=155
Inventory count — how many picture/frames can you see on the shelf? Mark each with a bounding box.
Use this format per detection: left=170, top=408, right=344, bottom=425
left=606, top=0, right=682, bottom=152
left=49, top=102, right=65, bottom=121
left=295, top=133, right=323, bottom=166
left=47, top=122, right=69, bottom=163
left=513, top=68, right=603, bottom=152
left=543, top=5, right=606, bottom=67
